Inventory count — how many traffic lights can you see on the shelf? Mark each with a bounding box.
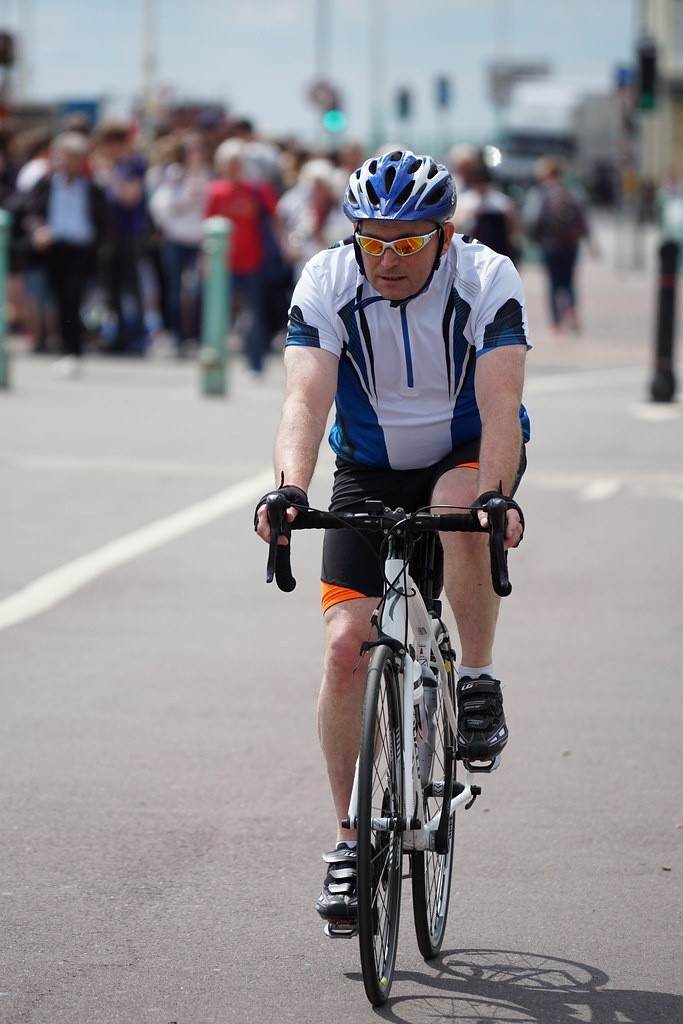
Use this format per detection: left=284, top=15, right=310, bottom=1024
left=321, top=109, right=348, bottom=135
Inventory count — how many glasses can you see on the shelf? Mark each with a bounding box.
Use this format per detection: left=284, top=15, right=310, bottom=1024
left=354, top=222, right=438, bottom=257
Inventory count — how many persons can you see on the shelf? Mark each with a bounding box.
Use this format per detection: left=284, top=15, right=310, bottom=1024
left=524, top=145, right=654, bottom=333
left=255, top=148, right=531, bottom=926
left=447, top=145, right=517, bottom=259
left=0, top=114, right=364, bottom=371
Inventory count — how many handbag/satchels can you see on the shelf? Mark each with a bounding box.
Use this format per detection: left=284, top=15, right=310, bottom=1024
left=258, top=190, right=280, bottom=281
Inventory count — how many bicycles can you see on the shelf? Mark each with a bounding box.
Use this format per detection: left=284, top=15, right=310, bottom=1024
left=250, top=500, right=512, bottom=1011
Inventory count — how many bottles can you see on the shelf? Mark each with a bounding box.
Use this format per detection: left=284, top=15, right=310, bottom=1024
left=408, top=644, right=428, bottom=745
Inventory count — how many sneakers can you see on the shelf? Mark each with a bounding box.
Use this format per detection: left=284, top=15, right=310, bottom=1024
left=456, top=674, right=509, bottom=761
left=316, top=843, right=362, bottom=920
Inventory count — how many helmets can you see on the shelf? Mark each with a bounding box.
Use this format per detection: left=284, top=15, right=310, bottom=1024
left=343, top=150, right=457, bottom=222
